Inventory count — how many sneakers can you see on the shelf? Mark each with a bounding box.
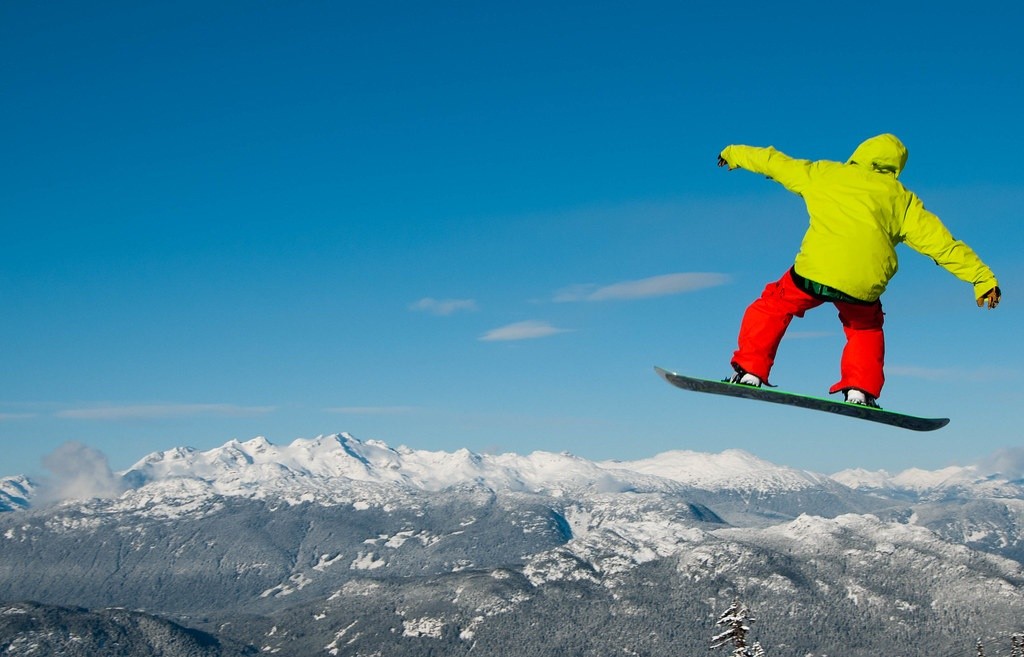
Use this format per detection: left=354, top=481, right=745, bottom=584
left=721, top=371, right=762, bottom=387
left=841, top=388, right=884, bottom=409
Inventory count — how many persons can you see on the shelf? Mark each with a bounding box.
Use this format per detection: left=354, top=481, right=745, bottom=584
left=717, top=131, right=1003, bottom=409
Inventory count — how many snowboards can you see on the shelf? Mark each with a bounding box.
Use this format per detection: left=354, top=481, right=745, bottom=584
left=654, top=364, right=950, bottom=432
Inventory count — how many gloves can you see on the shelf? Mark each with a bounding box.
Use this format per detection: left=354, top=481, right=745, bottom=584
left=717, top=154, right=740, bottom=172
left=976, top=286, right=1001, bottom=310
